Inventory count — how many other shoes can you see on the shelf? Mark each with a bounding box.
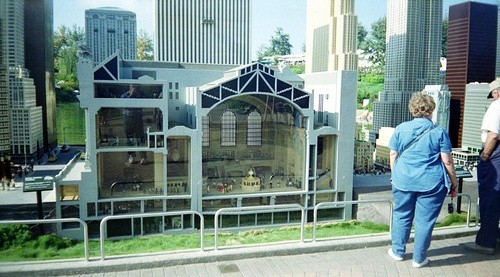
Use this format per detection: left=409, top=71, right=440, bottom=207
left=464, top=242, right=495, bottom=255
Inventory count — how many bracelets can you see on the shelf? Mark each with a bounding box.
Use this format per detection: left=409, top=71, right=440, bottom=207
left=450, top=184, right=457, bottom=192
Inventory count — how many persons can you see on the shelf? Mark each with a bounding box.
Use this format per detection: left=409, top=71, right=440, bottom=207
left=120, top=83, right=148, bottom=165
left=463, top=77, right=500, bottom=255
left=387, top=93, right=458, bottom=268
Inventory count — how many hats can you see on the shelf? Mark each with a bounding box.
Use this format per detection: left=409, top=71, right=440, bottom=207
left=487, top=78, right=500, bottom=99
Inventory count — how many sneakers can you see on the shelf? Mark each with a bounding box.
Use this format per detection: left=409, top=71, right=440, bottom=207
left=413, top=258, right=428, bottom=268
left=388, top=248, right=403, bottom=261
